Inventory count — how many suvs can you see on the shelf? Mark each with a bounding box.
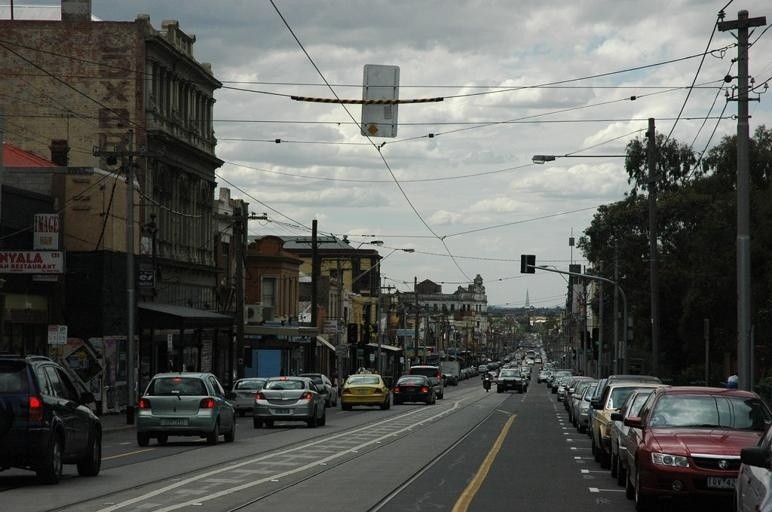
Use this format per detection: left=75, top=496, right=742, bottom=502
left=0, top=352, right=106, bottom=484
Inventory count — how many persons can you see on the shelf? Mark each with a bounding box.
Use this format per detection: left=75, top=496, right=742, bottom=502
left=728, top=371, right=738, bottom=387
left=358, top=363, right=366, bottom=371
left=481, top=369, right=493, bottom=383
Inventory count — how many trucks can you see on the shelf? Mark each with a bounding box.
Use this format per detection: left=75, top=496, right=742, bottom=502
left=439, top=360, right=460, bottom=386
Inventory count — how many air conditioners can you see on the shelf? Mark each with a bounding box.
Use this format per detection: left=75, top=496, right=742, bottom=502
left=246, top=303, right=265, bottom=324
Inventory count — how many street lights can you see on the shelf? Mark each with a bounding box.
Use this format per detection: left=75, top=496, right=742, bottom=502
left=342, top=247, right=414, bottom=393
left=531, top=153, right=662, bottom=380
left=336, top=237, right=383, bottom=386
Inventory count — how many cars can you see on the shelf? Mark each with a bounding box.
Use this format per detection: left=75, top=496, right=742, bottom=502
left=232, top=363, right=445, bottom=427
left=134, top=371, right=237, bottom=444
